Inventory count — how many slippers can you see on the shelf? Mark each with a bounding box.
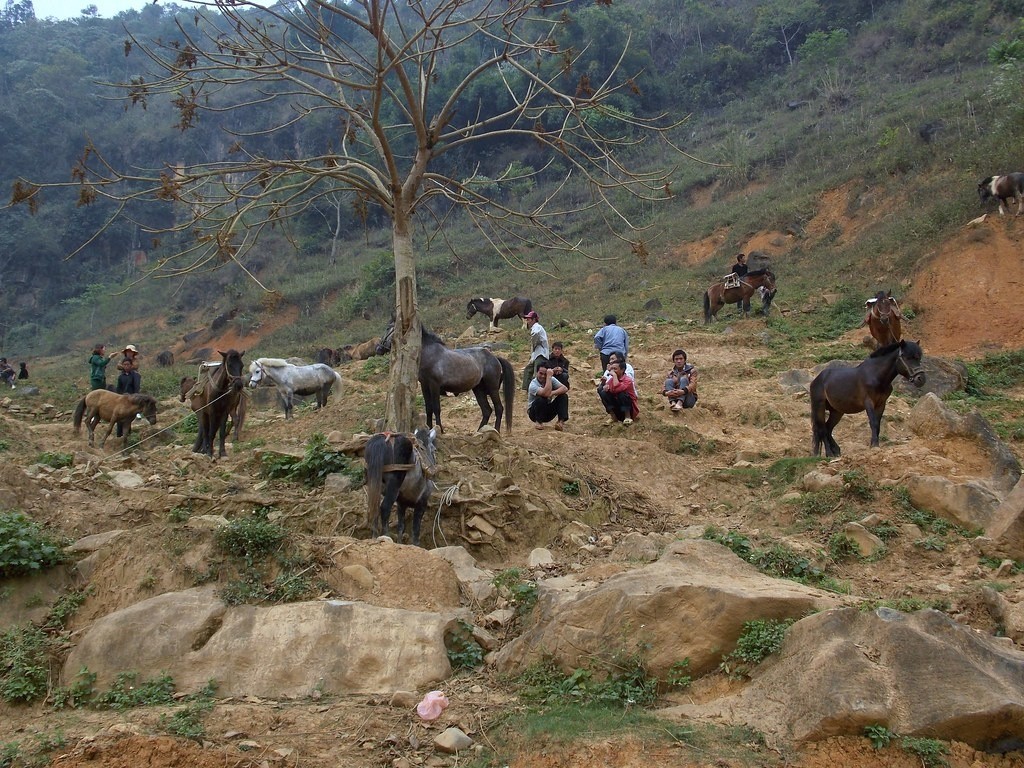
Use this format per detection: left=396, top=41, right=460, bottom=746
left=623, top=418, right=633, bottom=425
left=603, top=419, right=615, bottom=426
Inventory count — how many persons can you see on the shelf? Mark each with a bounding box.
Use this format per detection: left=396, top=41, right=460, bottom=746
left=18, top=362, right=28, bottom=379
left=732, top=254, right=750, bottom=316
left=600, top=360, right=639, bottom=425
left=0, top=358, right=16, bottom=389
left=662, top=350, right=698, bottom=410
left=527, top=363, right=569, bottom=430
left=117, top=345, right=141, bottom=419
left=549, top=342, right=570, bottom=390
left=88, top=344, right=118, bottom=422
left=978, top=181, right=989, bottom=204
left=594, top=315, right=628, bottom=371
left=597, top=351, right=638, bottom=397
left=522, top=311, right=549, bottom=391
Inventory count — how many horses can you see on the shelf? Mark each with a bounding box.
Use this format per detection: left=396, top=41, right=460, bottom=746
left=866, top=289, right=903, bottom=345
left=467, top=297, right=533, bottom=329
left=73, top=388, right=159, bottom=455
left=704, top=266, right=778, bottom=323
left=319, top=337, right=382, bottom=368
left=977, top=171, right=1023, bottom=216
left=374, top=313, right=515, bottom=433
left=179, top=349, right=247, bottom=457
left=246, top=357, right=344, bottom=418
left=809, top=339, right=924, bottom=458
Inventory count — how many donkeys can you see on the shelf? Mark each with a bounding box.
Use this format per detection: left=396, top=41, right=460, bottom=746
left=364, top=424, right=439, bottom=546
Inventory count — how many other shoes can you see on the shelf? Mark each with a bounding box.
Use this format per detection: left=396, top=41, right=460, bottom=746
left=535, top=423, right=544, bottom=429
left=137, top=414, right=142, bottom=420
left=670, top=403, right=683, bottom=411
left=11, top=385, right=15, bottom=389
left=556, top=423, right=563, bottom=431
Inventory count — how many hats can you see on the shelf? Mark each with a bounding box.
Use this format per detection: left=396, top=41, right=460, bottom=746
left=122, top=344, right=139, bottom=354
left=523, top=311, right=539, bottom=321
